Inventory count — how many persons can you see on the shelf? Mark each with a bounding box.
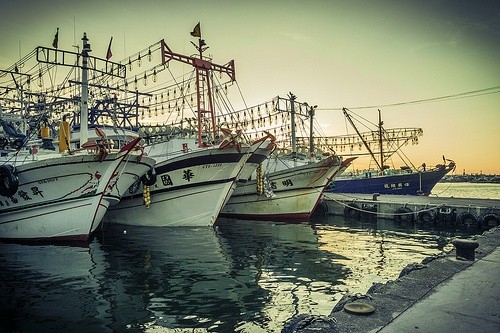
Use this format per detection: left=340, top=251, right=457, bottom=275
left=58, top=115, right=71, bottom=154
left=40, top=121, right=50, bottom=139
left=214, top=136, right=223, bottom=145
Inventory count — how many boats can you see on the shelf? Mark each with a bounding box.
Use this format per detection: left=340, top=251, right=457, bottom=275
left=0, top=32, right=457, bottom=245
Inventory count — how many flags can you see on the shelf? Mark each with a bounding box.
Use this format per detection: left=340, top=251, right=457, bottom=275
left=105, top=37, right=113, bottom=59
left=190, top=22, right=201, bottom=37
left=52, top=28, right=60, bottom=48
